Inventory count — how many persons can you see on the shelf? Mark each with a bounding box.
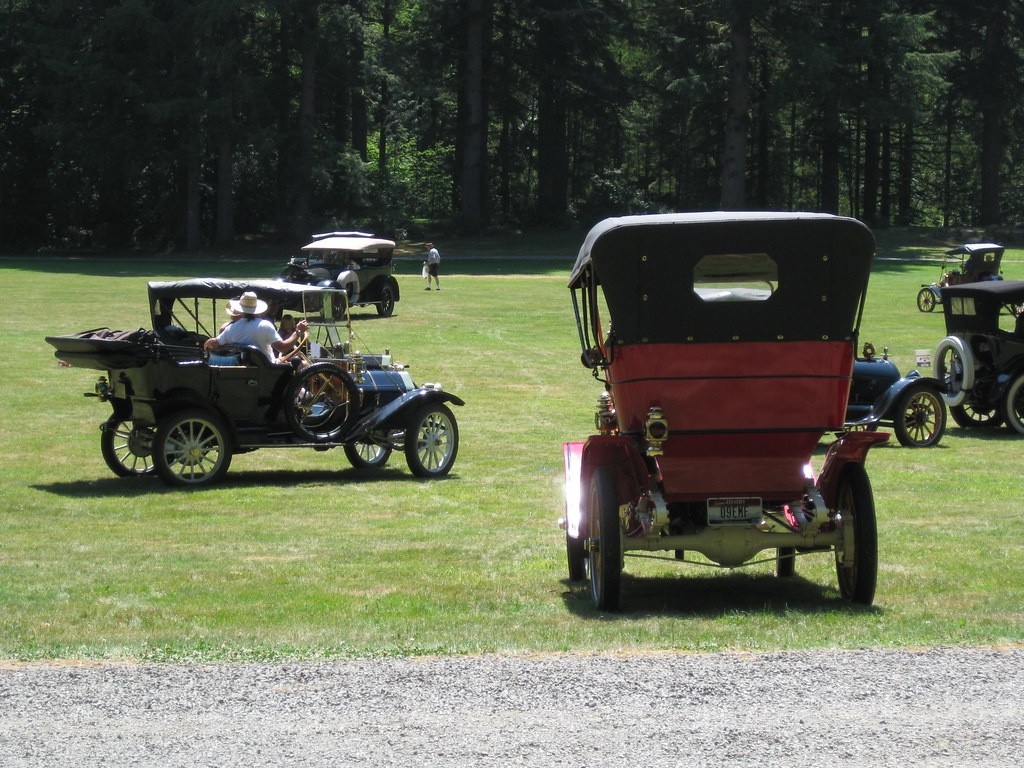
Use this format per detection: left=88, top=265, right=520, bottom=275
left=422, top=242, right=441, bottom=290
left=204, top=291, right=313, bottom=401
left=348, top=259, right=360, bottom=270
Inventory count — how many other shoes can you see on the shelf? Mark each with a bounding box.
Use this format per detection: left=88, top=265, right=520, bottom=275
left=301, top=392, right=326, bottom=407
left=436, top=289, right=440, bottom=290
left=425, top=287, right=431, bottom=290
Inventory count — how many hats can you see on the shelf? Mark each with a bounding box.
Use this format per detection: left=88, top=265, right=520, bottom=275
left=230, top=291, right=268, bottom=314
left=225, top=299, right=242, bottom=316
left=425, top=243, right=434, bottom=247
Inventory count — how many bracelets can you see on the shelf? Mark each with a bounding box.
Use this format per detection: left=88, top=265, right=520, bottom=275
left=294, top=329, right=301, bottom=337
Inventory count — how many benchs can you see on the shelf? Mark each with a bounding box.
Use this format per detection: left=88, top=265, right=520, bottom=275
left=224, top=345, right=290, bottom=401
left=207, top=349, right=249, bottom=366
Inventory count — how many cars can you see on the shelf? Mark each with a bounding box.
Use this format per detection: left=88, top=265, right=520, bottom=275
left=835, top=340, right=951, bottom=449
left=916, top=241, right=1008, bottom=314
left=270, top=229, right=401, bottom=322
left=932, top=277, right=1024, bottom=438
left=45, top=277, right=464, bottom=491
left=551, top=210, right=886, bottom=612
